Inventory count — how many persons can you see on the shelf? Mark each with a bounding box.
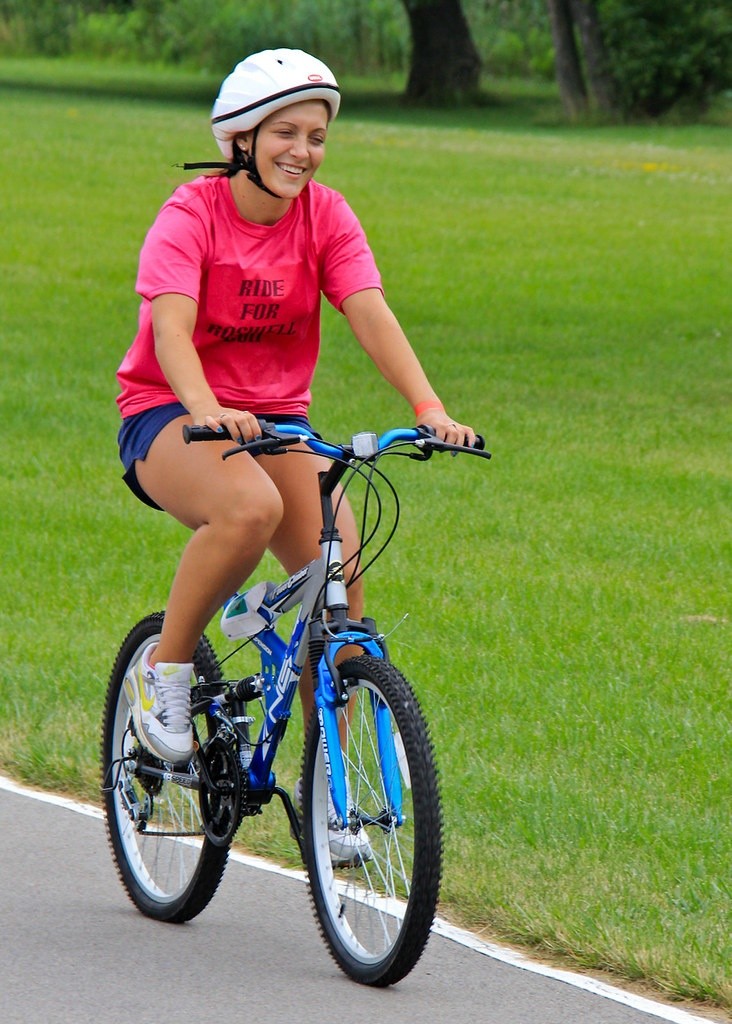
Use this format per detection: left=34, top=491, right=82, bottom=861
left=113, top=44, right=484, bottom=868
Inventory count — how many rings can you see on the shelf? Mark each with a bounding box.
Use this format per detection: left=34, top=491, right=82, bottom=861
left=221, top=413, right=228, bottom=422
left=450, top=423, right=458, bottom=428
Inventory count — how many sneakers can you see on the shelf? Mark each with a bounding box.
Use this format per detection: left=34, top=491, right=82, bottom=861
left=291, top=774, right=373, bottom=863
left=124, top=642, right=199, bottom=765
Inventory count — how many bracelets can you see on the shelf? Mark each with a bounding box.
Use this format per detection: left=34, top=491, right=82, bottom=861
left=413, top=402, right=446, bottom=417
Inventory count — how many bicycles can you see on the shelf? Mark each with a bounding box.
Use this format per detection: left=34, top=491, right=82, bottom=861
left=98, top=420, right=494, bottom=993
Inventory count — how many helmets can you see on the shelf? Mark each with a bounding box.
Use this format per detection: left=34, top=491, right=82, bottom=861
left=211, top=47, right=338, bottom=159
